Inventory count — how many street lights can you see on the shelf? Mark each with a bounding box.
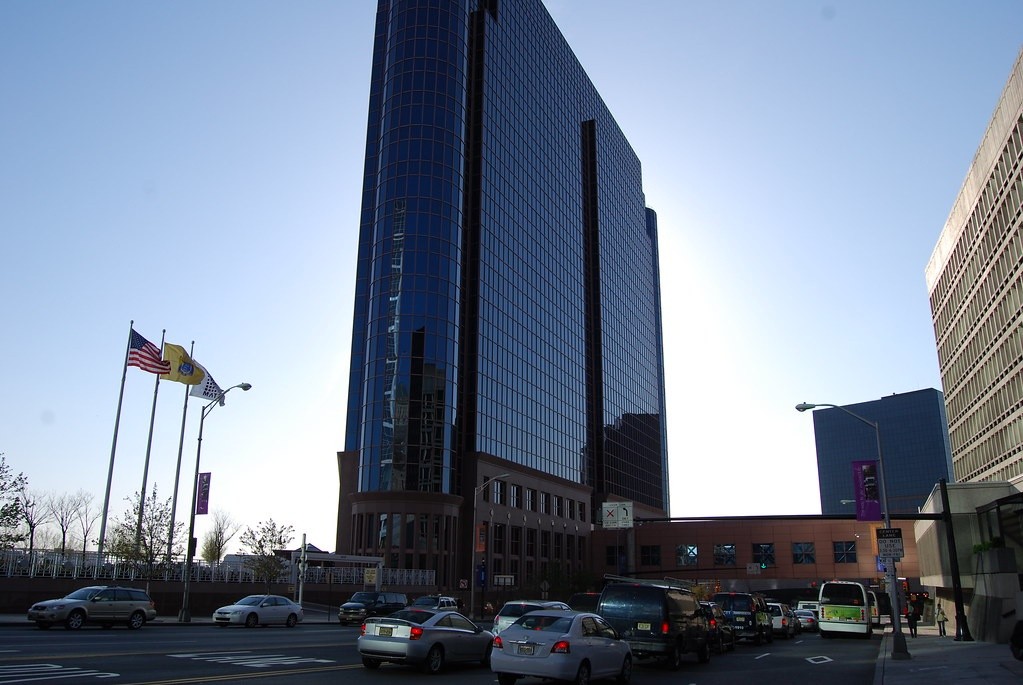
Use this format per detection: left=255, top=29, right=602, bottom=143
left=469, top=473, right=512, bottom=623
left=178, top=382, right=252, bottom=623
left=616, top=504, right=632, bottom=582
left=795, top=402, right=912, bottom=660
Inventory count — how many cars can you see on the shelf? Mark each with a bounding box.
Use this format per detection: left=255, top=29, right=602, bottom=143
left=793, top=609, right=819, bottom=632
left=212, top=594, right=304, bottom=629
left=356, top=607, right=495, bottom=675
left=492, top=600, right=575, bottom=636
left=490, top=609, right=633, bottom=685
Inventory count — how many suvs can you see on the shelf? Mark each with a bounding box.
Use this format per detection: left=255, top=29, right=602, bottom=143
left=27, top=585, right=156, bottom=631
left=696, top=601, right=735, bottom=654
left=758, top=601, right=795, bottom=640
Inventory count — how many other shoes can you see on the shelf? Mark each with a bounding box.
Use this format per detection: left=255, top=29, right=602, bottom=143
left=915, top=633, right=917, bottom=638
left=912, top=634, right=914, bottom=638
left=943, top=635, right=946, bottom=638
left=939, top=635, right=943, bottom=638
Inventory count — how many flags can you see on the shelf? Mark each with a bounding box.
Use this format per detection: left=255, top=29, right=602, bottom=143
left=128, top=329, right=171, bottom=374
left=190, top=361, right=225, bottom=406
left=161, top=342, right=205, bottom=385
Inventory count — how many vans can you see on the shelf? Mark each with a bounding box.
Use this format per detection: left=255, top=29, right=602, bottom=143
left=866, top=590, right=881, bottom=629
left=712, top=591, right=773, bottom=646
left=817, top=580, right=873, bottom=640
left=411, top=593, right=459, bottom=612
left=594, top=581, right=713, bottom=672
left=797, top=601, right=820, bottom=619
left=338, top=591, right=407, bottom=626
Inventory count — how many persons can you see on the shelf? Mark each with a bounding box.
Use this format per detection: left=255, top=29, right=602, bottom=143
left=457, top=599, right=463, bottom=611
left=903, top=599, right=921, bottom=638
left=934, top=604, right=948, bottom=638
